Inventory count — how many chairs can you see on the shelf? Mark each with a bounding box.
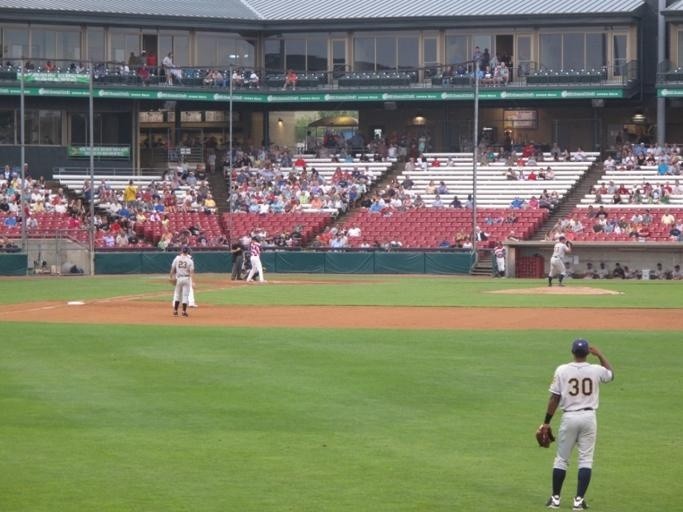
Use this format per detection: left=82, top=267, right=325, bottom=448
left=0, top=206, right=682, bottom=258
left=3, top=65, right=607, bottom=87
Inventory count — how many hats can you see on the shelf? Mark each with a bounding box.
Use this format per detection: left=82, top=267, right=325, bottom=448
left=571, top=339, right=589, bottom=354
left=182, top=245, right=190, bottom=252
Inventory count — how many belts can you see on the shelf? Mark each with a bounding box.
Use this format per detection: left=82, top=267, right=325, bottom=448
left=564, top=408, right=592, bottom=412
left=179, top=275, right=189, bottom=277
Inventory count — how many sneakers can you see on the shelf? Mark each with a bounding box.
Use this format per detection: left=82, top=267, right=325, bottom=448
left=572, top=496, right=586, bottom=511
left=172, top=311, right=189, bottom=317
left=544, top=495, right=560, bottom=509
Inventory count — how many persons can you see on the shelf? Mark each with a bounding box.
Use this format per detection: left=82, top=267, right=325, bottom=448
left=439, top=44, right=512, bottom=89
left=5, top=48, right=260, bottom=89
left=546, top=233, right=571, bottom=288
left=167, top=245, right=196, bottom=318
left=170, top=246, right=199, bottom=310
left=280, top=68, right=298, bottom=92
left=534, top=338, right=614, bottom=511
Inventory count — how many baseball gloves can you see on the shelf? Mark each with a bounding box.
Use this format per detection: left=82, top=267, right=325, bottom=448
left=535, top=423, right=555, bottom=448
left=565, top=241, right=572, bottom=249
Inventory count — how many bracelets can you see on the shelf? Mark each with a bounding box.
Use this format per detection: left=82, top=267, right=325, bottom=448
left=543, top=412, right=552, bottom=426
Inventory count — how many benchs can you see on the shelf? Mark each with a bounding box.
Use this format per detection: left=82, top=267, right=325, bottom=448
left=0, top=147, right=682, bottom=213
